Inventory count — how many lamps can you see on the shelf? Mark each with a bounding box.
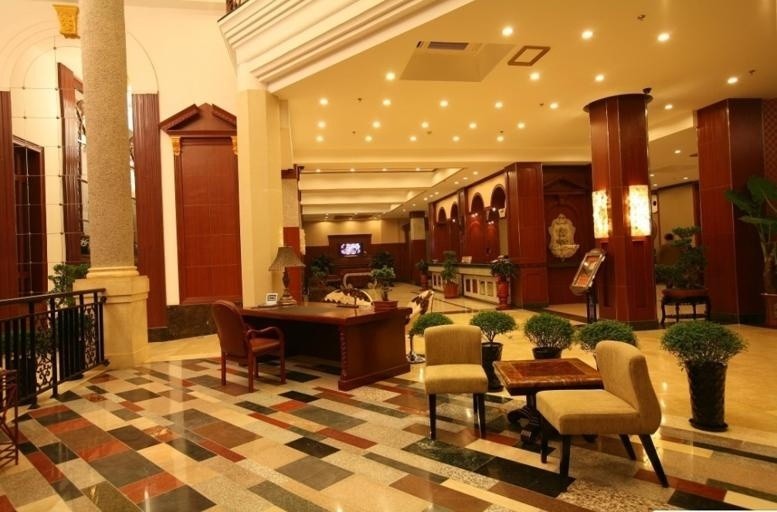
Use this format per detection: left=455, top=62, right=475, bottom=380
left=626, top=182, right=655, bottom=244
left=266, top=245, right=305, bottom=305
left=592, top=187, right=614, bottom=245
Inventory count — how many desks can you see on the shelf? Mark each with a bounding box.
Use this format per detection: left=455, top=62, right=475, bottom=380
left=0, top=367, right=18, bottom=471
left=240, top=298, right=413, bottom=391
left=660, top=296, right=713, bottom=326
left=492, top=356, right=609, bottom=451
left=337, top=267, right=379, bottom=291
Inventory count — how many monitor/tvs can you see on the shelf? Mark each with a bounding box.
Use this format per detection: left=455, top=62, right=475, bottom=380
left=340, top=242, right=362, bottom=257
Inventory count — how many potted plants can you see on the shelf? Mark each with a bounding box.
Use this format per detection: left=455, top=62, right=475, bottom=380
left=524, top=310, right=575, bottom=362
left=653, top=223, right=712, bottom=300
left=369, top=266, right=400, bottom=309
left=492, top=260, right=515, bottom=310
left=440, top=249, right=460, bottom=299
left=71, top=306, right=96, bottom=375
left=415, top=258, right=430, bottom=290
left=3, top=320, right=46, bottom=413
left=723, top=168, right=777, bottom=332
left=45, top=255, right=88, bottom=383
left=470, top=310, right=521, bottom=396
left=658, top=318, right=746, bottom=434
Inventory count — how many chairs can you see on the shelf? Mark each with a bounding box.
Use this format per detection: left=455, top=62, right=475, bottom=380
left=418, top=318, right=492, bottom=442
left=531, top=334, right=674, bottom=495
left=323, top=287, right=374, bottom=308
left=406, top=288, right=436, bottom=368
left=207, top=295, right=288, bottom=395
